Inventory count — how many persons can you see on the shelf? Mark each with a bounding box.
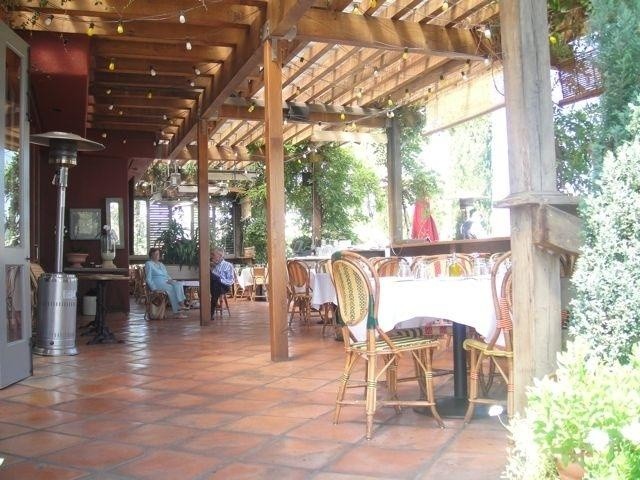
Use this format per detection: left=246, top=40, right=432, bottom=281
left=145, top=248, right=189, bottom=318
left=211, top=247, right=235, bottom=320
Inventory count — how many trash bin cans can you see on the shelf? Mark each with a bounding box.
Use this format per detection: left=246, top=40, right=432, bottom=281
left=82, top=295, right=97, bottom=316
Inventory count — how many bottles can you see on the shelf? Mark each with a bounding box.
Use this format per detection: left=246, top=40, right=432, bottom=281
left=449, top=256, right=461, bottom=279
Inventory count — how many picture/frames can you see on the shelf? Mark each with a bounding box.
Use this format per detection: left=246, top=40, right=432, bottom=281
left=69, top=209, right=102, bottom=241
left=106, top=197, right=125, bottom=250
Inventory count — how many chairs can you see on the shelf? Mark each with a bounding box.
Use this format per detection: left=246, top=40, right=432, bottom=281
left=368, top=250, right=512, bottom=277
left=132, top=255, right=200, bottom=320
left=414, top=253, right=478, bottom=396
left=462, top=251, right=515, bottom=425
left=212, top=261, right=269, bottom=318
left=485, top=251, right=512, bottom=391
left=369, top=256, right=425, bottom=413
left=28, top=262, right=46, bottom=289
left=331, top=252, right=447, bottom=440
left=286, top=256, right=344, bottom=337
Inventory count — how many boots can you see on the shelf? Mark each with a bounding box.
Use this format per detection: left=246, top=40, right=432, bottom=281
left=211, top=307, right=216, bottom=320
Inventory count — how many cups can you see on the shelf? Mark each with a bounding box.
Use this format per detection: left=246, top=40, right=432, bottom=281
left=472, top=258, right=488, bottom=278
left=396, top=260, right=413, bottom=280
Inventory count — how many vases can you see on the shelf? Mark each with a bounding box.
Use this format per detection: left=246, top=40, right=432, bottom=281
left=101, top=236, right=116, bottom=269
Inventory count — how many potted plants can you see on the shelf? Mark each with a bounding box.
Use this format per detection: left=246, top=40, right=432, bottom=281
left=487, top=337, right=640, bottom=480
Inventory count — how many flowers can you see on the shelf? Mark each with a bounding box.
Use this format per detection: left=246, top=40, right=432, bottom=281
left=102, top=225, right=118, bottom=240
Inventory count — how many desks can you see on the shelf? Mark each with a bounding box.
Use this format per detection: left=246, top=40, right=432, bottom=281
left=63, top=267, right=131, bottom=346
left=347, top=274, right=510, bottom=420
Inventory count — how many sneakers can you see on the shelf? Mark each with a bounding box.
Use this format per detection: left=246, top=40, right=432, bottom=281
left=173, top=307, right=190, bottom=314
